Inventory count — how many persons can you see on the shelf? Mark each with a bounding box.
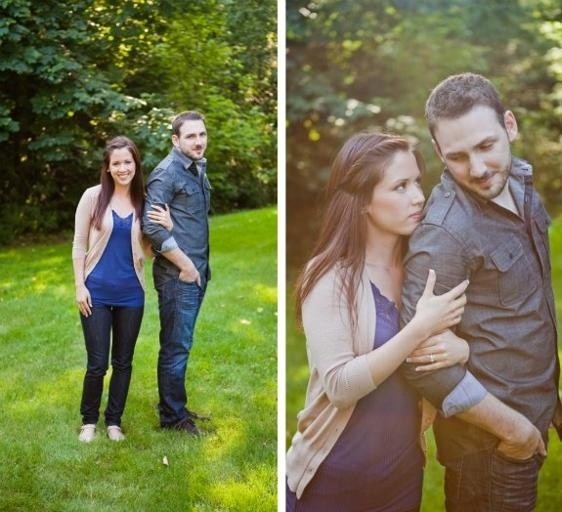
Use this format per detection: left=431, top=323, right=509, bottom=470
left=71, top=135, right=174, bottom=444
left=399, top=72, right=562, bottom=512
left=142, top=110, right=218, bottom=435
left=285, top=131, right=470, bottom=511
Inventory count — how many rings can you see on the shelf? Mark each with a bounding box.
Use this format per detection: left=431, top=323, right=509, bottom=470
left=430, top=353, right=435, bottom=364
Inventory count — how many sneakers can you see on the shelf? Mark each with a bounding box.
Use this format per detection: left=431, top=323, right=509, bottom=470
left=160, top=417, right=201, bottom=438
left=184, top=408, right=210, bottom=422
left=78, top=422, right=97, bottom=443
left=105, top=423, right=125, bottom=441
